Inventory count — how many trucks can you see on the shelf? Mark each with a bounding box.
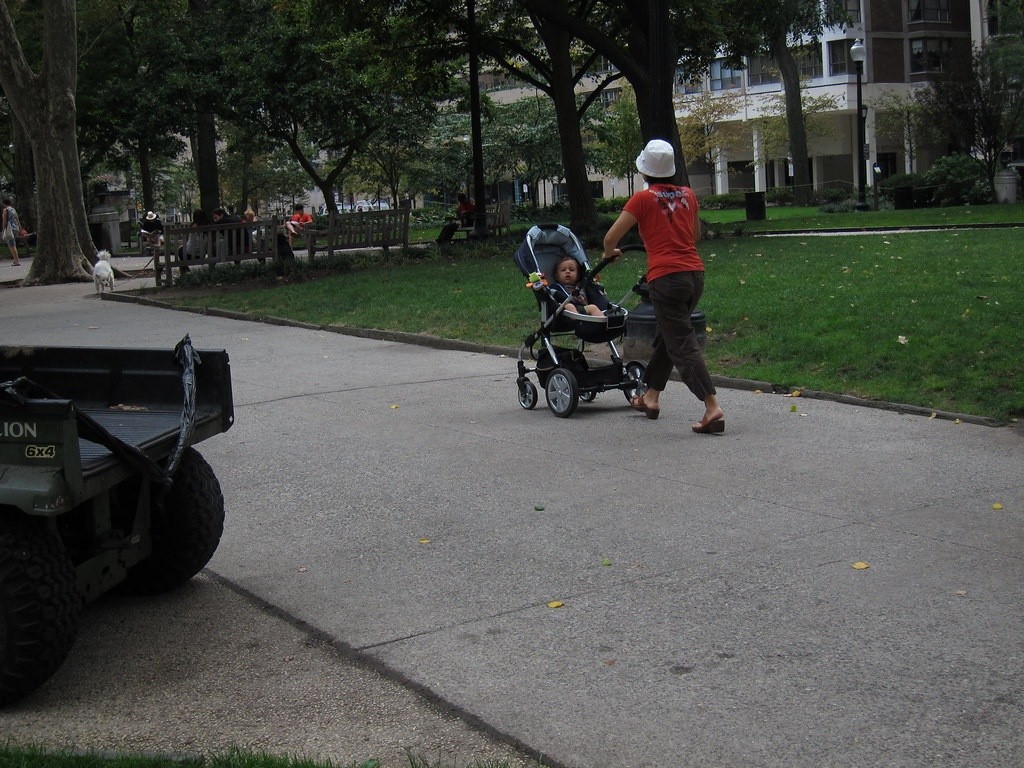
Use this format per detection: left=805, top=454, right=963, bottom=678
left=0, top=346, right=236, bottom=702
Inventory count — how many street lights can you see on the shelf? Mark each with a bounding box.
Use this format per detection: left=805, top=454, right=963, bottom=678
left=851, top=37, right=870, bottom=210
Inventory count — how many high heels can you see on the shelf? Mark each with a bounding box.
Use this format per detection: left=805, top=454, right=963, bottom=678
left=692, top=414, right=725, bottom=433
left=629, top=395, right=660, bottom=419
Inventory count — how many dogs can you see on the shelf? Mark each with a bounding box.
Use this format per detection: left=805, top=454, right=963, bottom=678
left=93, top=250, right=114, bottom=295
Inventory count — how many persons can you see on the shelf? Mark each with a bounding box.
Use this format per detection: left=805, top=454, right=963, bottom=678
left=604, top=139, right=724, bottom=433
left=177, top=209, right=211, bottom=280
left=244, top=209, right=265, bottom=248
left=136, top=211, right=165, bottom=249
left=210, top=208, right=249, bottom=270
left=548, top=257, right=605, bottom=316
left=0, top=199, right=27, bottom=266
left=435, top=194, right=475, bottom=239
left=284, top=204, right=313, bottom=248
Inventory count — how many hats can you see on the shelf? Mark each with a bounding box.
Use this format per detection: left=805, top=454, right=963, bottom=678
left=636, top=140, right=676, bottom=178
left=146, top=211, right=156, bottom=220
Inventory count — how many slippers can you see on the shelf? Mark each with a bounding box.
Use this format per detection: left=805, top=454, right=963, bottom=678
left=11, top=262, right=20, bottom=266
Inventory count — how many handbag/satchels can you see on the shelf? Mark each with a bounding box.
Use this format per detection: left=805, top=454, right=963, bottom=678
left=2, top=221, right=14, bottom=240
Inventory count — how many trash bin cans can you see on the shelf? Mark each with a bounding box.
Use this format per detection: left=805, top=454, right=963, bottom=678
left=743, top=191, right=766, bottom=220
left=894, top=186, right=913, bottom=209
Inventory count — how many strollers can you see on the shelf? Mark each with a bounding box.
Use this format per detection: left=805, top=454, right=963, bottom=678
left=513, top=223, right=650, bottom=418
